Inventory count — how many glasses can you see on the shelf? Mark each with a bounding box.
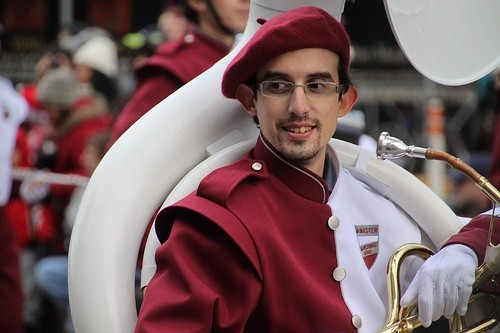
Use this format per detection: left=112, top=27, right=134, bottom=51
left=254, top=81, right=346, bottom=98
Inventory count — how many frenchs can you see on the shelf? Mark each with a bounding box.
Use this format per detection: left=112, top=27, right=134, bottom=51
left=66, top=0, right=500, bottom=331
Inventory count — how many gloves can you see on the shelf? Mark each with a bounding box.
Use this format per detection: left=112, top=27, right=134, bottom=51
left=400, top=244, right=478, bottom=328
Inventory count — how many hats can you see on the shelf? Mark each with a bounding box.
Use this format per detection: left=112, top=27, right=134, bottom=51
left=222, top=6, right=351, bottom=99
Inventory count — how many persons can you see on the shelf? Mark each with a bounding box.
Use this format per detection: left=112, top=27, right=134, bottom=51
left=133, top=6, right=500, bottom=333
left=103, top=0, right=251, bottom=158
left=0, top=28, right=118, bottom=333
